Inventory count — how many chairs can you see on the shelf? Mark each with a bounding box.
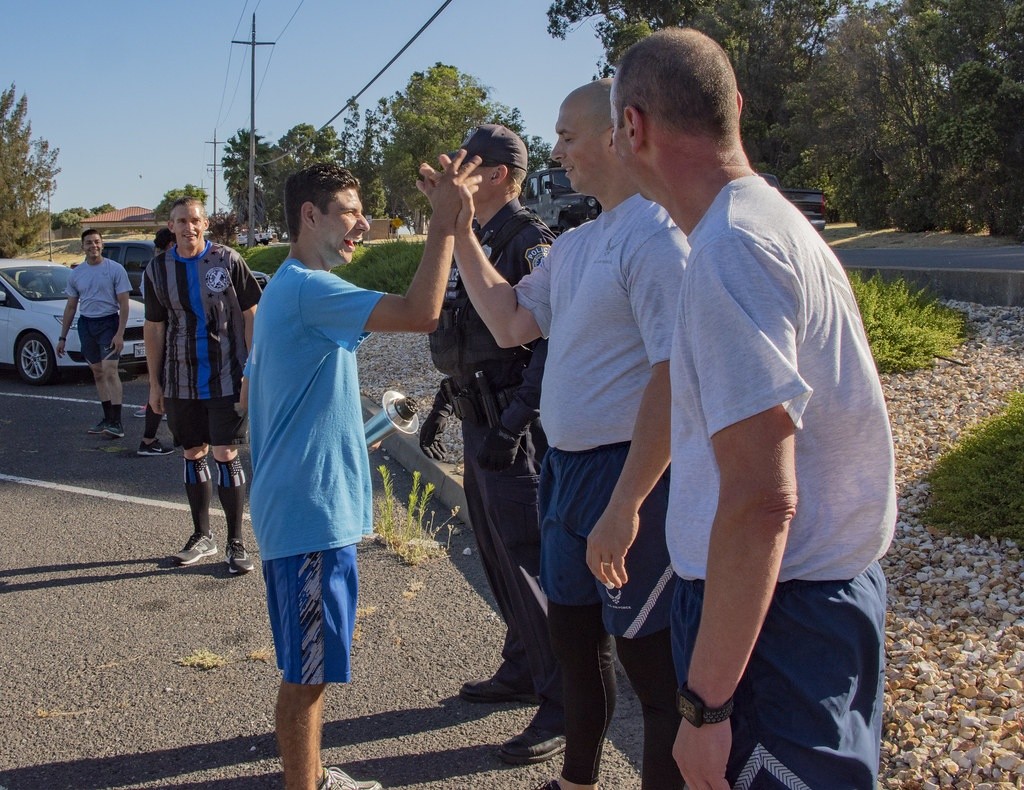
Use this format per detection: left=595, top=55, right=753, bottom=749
left=19, top=273, right=35, bottom=287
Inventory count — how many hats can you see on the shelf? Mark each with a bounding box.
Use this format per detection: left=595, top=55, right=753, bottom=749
left=444, top=124, right=528, bottom=170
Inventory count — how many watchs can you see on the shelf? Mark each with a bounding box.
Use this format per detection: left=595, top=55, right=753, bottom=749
left=59, top=337, right=66, bottom=342
left=674, top=680, right=735, bottom=728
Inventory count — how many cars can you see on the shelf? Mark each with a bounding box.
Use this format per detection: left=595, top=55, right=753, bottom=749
left=0, top=258, right=149, bottom=387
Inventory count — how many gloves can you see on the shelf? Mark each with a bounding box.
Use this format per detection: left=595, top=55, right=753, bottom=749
left=419, top=409, right=448, bottom=461
left=476, top=419, right=521, bottom=472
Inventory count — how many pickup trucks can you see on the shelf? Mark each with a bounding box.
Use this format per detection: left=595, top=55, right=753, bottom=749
left=71, top=240, right=271, bottom=304
left=237, top=229, right=274, bottom=247
left=522, top=167, right=601, bottom=237
left=755, top=173, right=826, bottom=232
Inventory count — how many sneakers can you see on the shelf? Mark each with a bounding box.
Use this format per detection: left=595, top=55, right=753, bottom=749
left=316, top=767, right=382, bottom=790
left=88, top=418, right=124, bottom=438
left=459, top=677, right=543, bottom=705
left=172, top=533, right=218, bottom=565
left=162, top=414, right=168, bottom=421
left=137, top=437, right=174, bottom=456
left=498, top=723, right=566, bottom=765
left=225, top=538, right=253, bottom=573
left=531, top=780, right=563, bottom=790
left=134, top=404, right=147, bottom=417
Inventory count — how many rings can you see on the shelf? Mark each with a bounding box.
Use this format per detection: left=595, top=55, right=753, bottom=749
left=603, top=562, right=611, bottom=566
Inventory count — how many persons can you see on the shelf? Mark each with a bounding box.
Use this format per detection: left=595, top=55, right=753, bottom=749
left=611, top=26, right=897, bottom=790
left=134, top=227, right=177, bottom=456
left=240, top=149, right=482, bottom=790
left=143, top=197, right=263, bottom=575
left=420, top=78, right=691, bottom=790
left=56, top=229, right=134, bottom=438
left=418, top=125, right=566, bottom=764
